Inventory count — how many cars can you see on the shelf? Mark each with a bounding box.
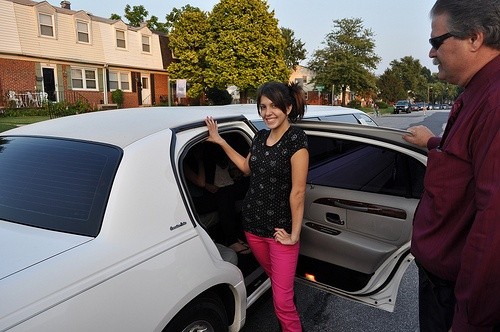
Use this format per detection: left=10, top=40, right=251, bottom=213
left=411, top=102, right=450, bottom=112
left=0, top=100, right=439, bottom=332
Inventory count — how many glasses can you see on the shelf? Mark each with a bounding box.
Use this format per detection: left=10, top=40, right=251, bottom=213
left=428, top=32, right=453, bottom=50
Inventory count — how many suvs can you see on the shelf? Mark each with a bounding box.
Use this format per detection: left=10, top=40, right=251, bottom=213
left=394, top=100, right=412, bottom=114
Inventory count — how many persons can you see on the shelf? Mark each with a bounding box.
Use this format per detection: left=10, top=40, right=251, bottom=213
left=204, top=80, right=311, bottom=332
left=401, top=1, right=500, bottom=332
